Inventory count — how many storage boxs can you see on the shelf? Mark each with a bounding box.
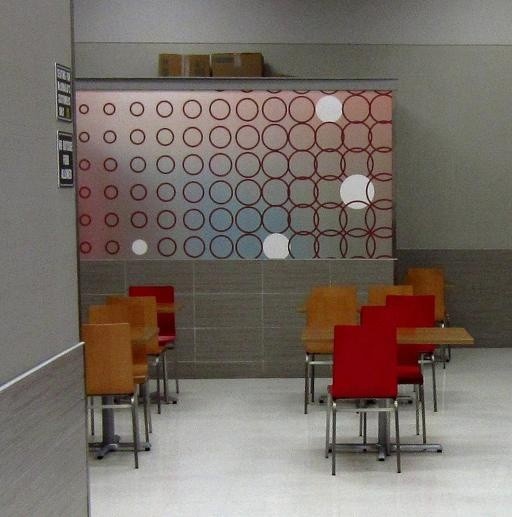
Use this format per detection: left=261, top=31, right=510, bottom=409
left=158, top=53, right=266, bottom=77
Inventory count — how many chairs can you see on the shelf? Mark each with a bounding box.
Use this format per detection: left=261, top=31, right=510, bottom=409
left=81, top=285, right=187, bottom=469
left=295, top=268, right=474, bottom=476
